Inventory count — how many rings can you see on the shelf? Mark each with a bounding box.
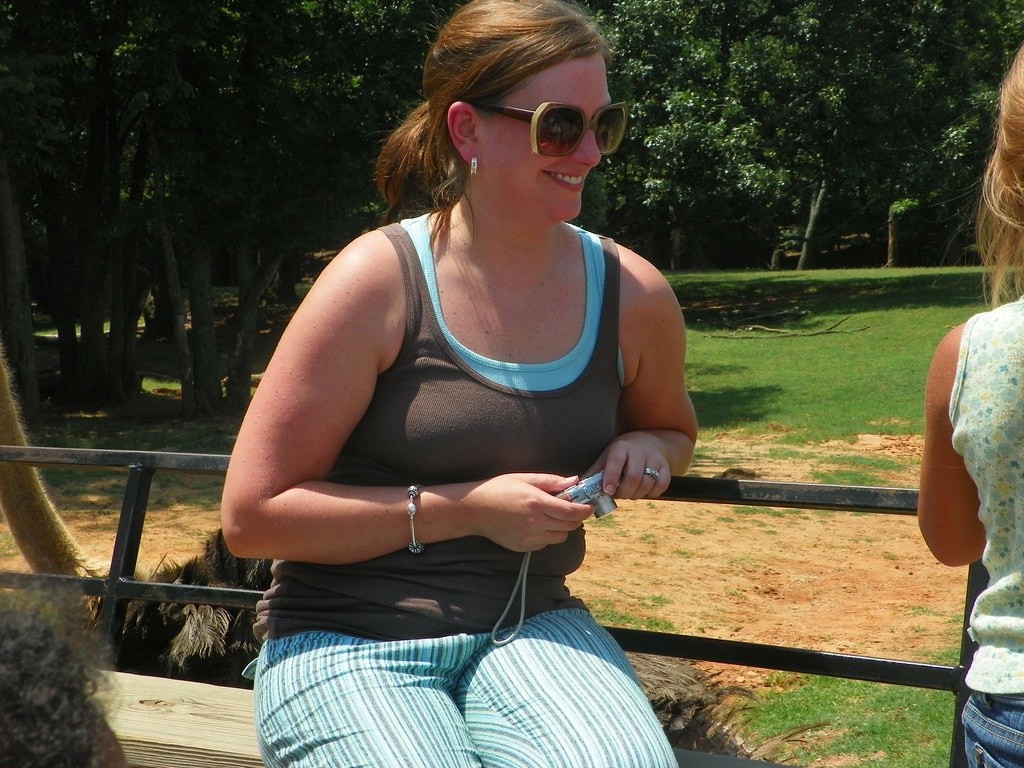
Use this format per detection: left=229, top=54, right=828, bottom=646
left=643, top=468, right=660, bottom=481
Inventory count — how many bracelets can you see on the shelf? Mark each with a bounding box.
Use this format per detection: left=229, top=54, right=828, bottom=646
left=406, top=483, right=425, bottom=555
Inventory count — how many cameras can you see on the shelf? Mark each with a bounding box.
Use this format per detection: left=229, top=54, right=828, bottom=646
left=554, top=470, right=621, bottom=518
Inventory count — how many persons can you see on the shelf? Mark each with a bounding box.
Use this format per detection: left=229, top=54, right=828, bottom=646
left=0, top=582, right=128, bottom=768
left=218, top=0, right=701, bottom=768
left=914, top=44, right=1024, bottom=768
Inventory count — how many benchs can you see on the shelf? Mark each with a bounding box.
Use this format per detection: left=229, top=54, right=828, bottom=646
left=0, top=445, right=992, bottom=768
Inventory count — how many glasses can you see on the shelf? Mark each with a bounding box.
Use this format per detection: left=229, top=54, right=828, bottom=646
left=477, top=102, right=628, bottom=157
